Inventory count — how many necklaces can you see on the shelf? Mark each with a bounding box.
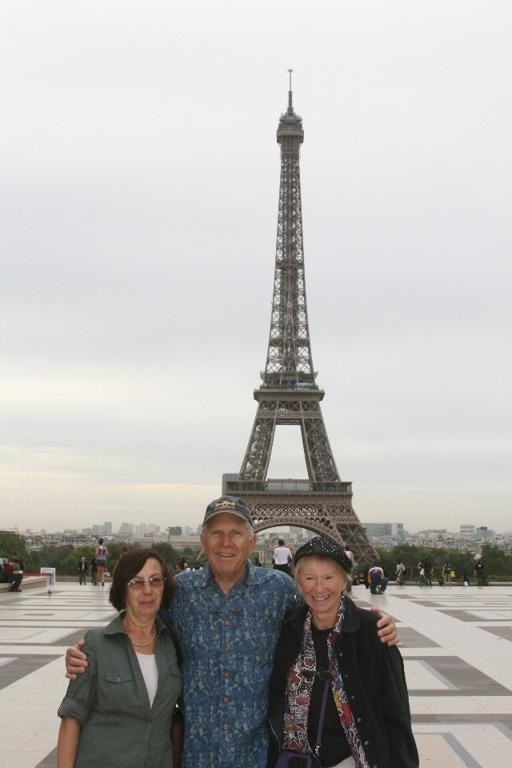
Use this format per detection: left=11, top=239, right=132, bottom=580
left=129, top=623, right=157, bottom=647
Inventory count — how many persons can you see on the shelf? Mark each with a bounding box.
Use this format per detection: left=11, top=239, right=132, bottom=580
left=343, top=544, right=489, bottom=594
left=3, top=557, right=24, bottom=593
left=65, top=497, right=400, bottom=767
left=78, top=538, right=205, bottom=586
left=56, top=547, right=182, bottom=767
left=265, top=536, right=419, bottom=767
left=273, top=539, right=293, bottom=574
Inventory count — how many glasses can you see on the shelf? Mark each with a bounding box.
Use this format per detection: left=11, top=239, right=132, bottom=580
left=126, top=574, right=167, bottom=589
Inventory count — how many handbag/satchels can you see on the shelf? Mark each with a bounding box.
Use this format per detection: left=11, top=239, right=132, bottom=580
left=274, top=752, right=321, bottom=767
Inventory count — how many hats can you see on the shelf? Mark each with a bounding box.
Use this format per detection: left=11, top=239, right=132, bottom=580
left=293, top=536, right=353, bottom=574
left=202, top=496, right=256, bottom=529
